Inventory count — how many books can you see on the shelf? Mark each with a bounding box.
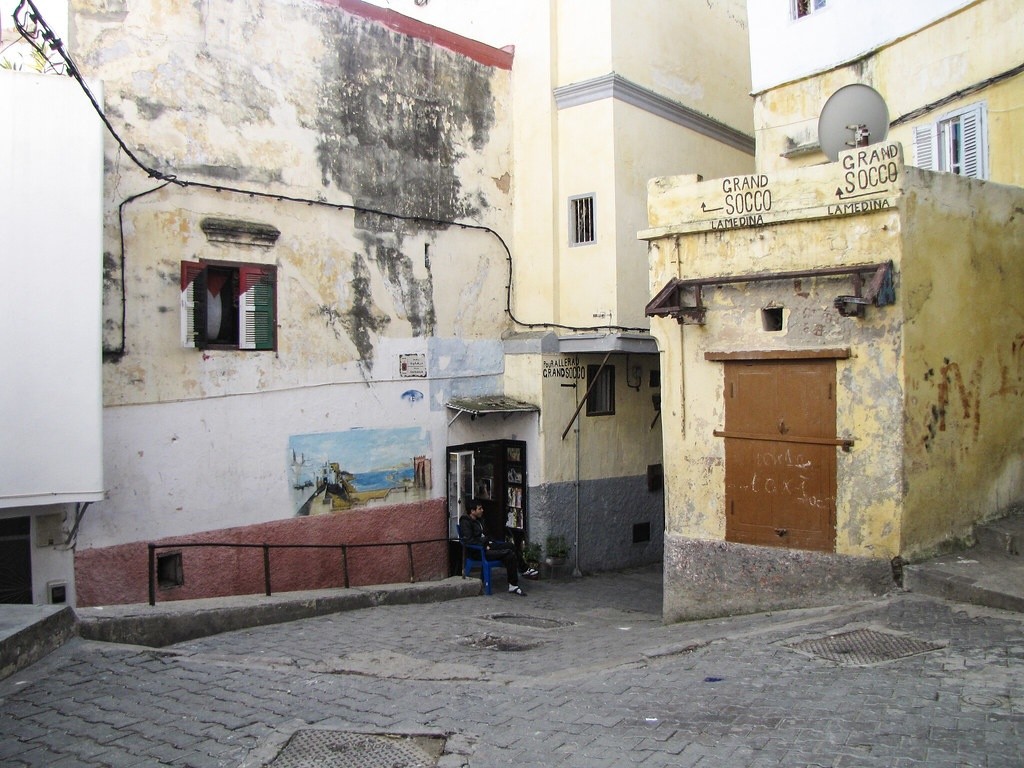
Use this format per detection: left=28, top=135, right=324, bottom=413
left=477, top=477, right=492, bottom=500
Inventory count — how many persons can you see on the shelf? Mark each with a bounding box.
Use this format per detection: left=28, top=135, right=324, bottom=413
left=509, top=448, right=520, bottom=461
left=482, top=481, right=487, bottom=496
left=509, top=468, right=518, bottom=482
left=459, top=502, right=539, bottom=596
left=510, top=488, right=515, bottom=506
left=506, top=508, right=515, bottom=528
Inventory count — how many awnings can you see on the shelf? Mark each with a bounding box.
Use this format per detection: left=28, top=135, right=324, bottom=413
left=446, top=395, right=538, bottom=427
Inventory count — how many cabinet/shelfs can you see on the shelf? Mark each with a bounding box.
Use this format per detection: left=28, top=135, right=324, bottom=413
left=448, top=452, right=475, bottom=541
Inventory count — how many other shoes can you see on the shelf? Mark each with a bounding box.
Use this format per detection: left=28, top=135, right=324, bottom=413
left=522, top=568, right=539, bottom=577
left=509, top=586, right=527, bottom=596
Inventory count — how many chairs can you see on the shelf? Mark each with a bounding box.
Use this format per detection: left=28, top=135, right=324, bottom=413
left=456, top=524, right=505, bottom=596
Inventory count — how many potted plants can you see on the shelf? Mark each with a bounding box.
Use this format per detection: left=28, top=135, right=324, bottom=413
left=523, top=541, right=541, bottom=582
left=544, top=534, right=572, bottom=565
